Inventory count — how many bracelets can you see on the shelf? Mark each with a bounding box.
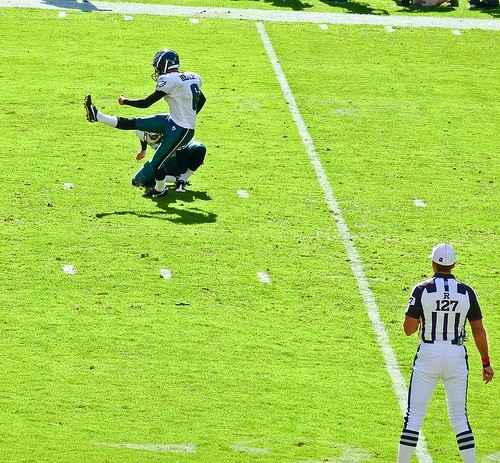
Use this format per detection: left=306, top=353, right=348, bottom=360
left=481, top=356, right=490, bottom=368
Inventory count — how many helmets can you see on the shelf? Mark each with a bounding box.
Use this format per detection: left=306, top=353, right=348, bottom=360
left=145, top=131, right=163, bottom=144
left=151, top=49, right=180, bottom=74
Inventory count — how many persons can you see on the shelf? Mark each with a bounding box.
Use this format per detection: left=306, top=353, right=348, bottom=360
left=84, top=48, right=207, bottom=198
left=397, top=243, right=494, bottom=462
left=131, top=129, right=206, bottom=194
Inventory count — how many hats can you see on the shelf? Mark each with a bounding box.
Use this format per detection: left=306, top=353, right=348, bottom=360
left=429, top=243, right=455, bottom=266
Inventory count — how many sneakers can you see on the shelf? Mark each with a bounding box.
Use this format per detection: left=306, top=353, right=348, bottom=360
left=84, top=95, right=98, bottom=122
left=185, top=180, right=192, bottom=186
left=142, top=185, right=168, bottom=199
left=175, top=180, right=185, bottom=192
